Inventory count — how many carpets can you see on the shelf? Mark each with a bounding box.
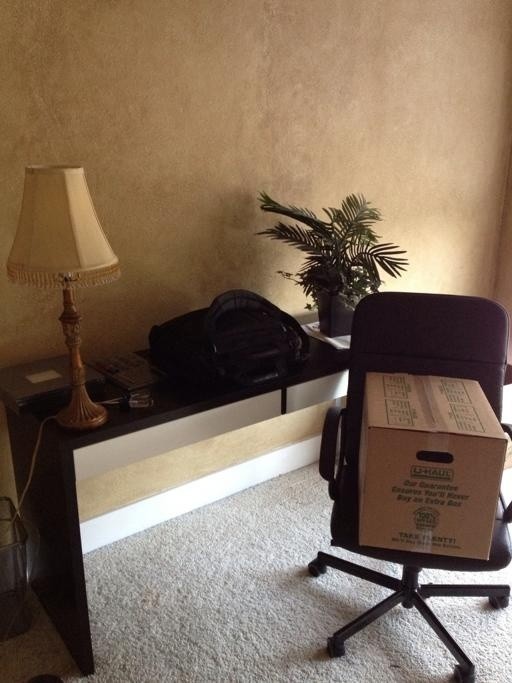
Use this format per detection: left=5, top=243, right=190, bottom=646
left=83, top=462, right=511, bottom=682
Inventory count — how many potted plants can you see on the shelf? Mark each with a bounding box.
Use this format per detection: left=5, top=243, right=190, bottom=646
left=252, top=187, right=407, bottom=337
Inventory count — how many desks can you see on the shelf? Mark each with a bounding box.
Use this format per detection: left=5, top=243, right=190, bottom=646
left=2, top=312, right=352, bottom=674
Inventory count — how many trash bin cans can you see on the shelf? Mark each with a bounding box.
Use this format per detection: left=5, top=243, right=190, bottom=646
left=0, top=497, right=32, bottom=643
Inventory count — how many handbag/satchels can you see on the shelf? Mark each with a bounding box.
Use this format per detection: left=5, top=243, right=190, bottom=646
left=148, top=289, right=312, bottom=389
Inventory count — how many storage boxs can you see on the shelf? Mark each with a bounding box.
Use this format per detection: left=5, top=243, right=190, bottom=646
left=357, top=373, right=507, bottom=562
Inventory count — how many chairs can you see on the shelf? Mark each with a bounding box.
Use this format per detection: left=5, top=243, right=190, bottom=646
left=308, top=292, right=511, bottom=683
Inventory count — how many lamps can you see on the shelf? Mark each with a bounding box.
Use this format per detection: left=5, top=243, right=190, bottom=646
left=4, top=163, right=120, bottom=435
